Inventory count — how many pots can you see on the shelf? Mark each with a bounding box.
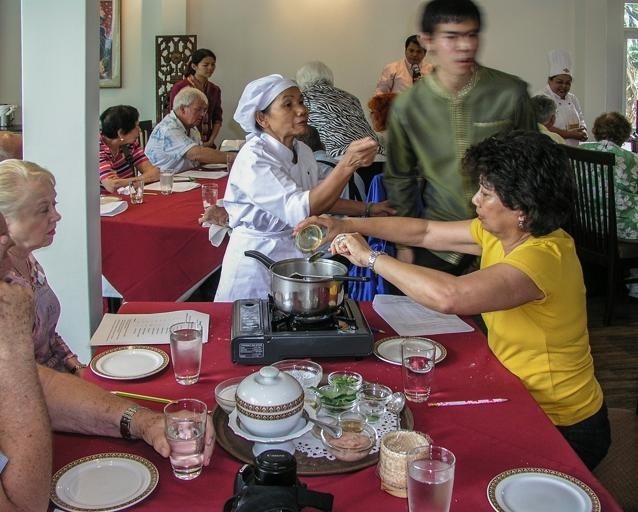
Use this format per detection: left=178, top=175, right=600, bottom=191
left=245, top=248, right=349, bottom=318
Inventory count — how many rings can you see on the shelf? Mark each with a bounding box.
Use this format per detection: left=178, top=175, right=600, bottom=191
left=337, top=235, right=345, bottom=241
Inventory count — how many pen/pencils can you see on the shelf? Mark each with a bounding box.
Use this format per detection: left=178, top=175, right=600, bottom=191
left=110, top=391, right=171, bottom=404
left=370, top=328, right=385, bottom=333
left=428, top=398, right=507, bottom=407
left=143, top=193, right=158, bottom=195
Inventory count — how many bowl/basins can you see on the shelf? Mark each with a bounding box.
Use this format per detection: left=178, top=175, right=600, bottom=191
left=307, top=370, right=392, bottom=438
left=269, top=360, right=324, bottom=388
left=322, top=422, right=379, bottom=459
left=213, top=376, right=244, bottom=413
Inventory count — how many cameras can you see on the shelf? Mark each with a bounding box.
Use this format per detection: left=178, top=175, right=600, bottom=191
left=233, top=449, right=298, bottom=512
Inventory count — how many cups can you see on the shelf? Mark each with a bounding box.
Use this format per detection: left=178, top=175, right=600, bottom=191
left=227, top=153, right=239, bottom=176
left=296, top=225, right=321, bottom=252
left=162, top=398, right=208, bottom=483
left=378, top=431, right=430, bottom=499
left=398, top=338, right=436, bottom=404
left=158, top=168, right=173, bottom=196
left=201, top=182, right=218, bottom=210
left=127, top=181, right=145, bottom=204
left=168, top=321, right=202, bottom=387
left=400, top=445, right=456, bottom=512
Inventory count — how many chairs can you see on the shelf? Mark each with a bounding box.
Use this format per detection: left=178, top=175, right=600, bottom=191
left=134, top=120, right=152, bottom=175
left=563, top=145, right=638, bottom=325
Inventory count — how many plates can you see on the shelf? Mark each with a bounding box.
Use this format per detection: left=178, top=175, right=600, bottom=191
left=219, top=405, right=317, bottom=444
left=88, top=344, right=170, bottom=381
left=173, top=176, right=195, bottom=183
left=200, top=163, right=227, bottom=170
left=51, top=452, right=159, bottom=512
left=100, top=195, right=122, bottom=207
left=486, top=467, right=601, bottom=512
left=372, top=335, right=450, bottom=366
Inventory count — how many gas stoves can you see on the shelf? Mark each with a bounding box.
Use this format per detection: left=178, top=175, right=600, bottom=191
left=230, top=296, right=375, bottom=367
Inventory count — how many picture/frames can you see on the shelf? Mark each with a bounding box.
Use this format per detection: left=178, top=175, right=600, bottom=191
left=100, top=1, right=122, bottom=89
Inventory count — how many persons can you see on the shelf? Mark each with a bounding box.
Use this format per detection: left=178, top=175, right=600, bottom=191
left=0, top=158, right=88, bottom=379
left=102, top=105, right=160, bottom=193
left=568, top=112, right=636, bottom=297
left=213, top=75, right=398, bottom=302
left=138, top=86, right=238, bottom=176
left=198, top=205, right=233, bottom=229
left=383, top=1, right=540, bottom=337
left=0, top=211, right=216, bottom=512
left=368, top=93, right=400, bottom=139
left=291, top=129, right=612, bottom=470
left=296, top=61, right=387, bottom=196
left=376, top=34, right=435, bottom=93
left=532, top=49, right=588, bottom=148
left=295, top=126, right=367, bottom=202
left=168, top=48, right=223, bottom=149
left=530, top=95, right=571, bottom=166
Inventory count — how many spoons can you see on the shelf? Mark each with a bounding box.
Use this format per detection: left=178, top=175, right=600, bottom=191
left=387, top=390, right=406, bottom=429
left=301, top=410, right=343, bottom=439
left=305, top=249, right=332, bottom=263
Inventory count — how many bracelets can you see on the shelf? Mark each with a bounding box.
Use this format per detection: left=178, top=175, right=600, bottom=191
left=366, top=201, right=374, bottom=217
left=368, top=251, right=387, bottom=274
left=224, top=212, right=231, bottom=228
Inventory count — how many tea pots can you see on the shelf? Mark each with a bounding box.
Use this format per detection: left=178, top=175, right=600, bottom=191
left=0, top=104, right=17, bottom=131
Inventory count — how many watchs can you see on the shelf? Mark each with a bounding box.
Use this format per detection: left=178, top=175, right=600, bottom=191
left=70, top=364, right=87, bottom=375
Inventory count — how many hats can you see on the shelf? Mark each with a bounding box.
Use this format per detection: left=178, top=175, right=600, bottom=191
left=548, top=48, right=573, bottom=82
left=233, top=73, right=298, bottom=134
